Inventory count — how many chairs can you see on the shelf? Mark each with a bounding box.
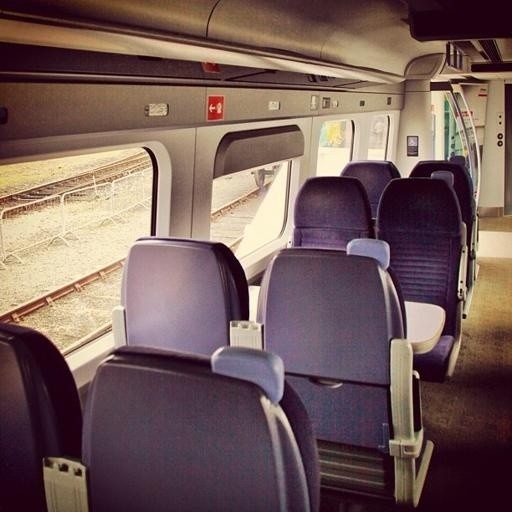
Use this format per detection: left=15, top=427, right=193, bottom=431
left=0, top=323, right=322, bottom=511
left=294, top=158, right=480, bottom=382
left=111, top=236, right=446, bottom=511
